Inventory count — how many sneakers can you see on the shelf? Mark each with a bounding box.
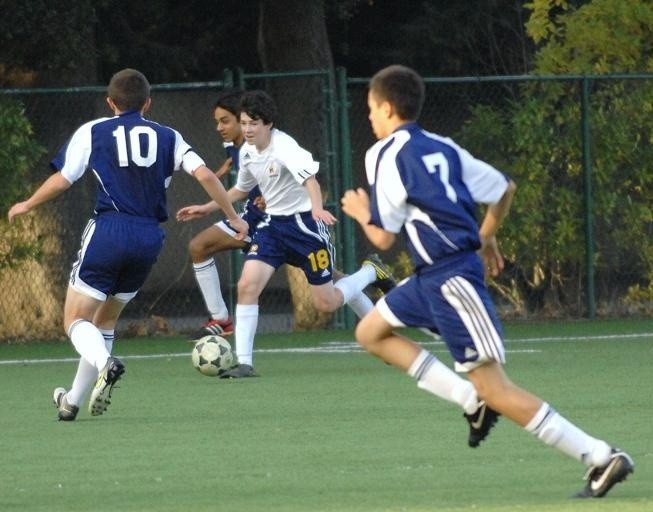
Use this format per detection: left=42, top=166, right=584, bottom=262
left=574, top=448, right=635, bottom=501
left=464, top=397, right=500, bottom=447
left=220, top=363, right=258, bottom=378
left=187, top=315, right=234, bottom=343
left=362, top=253, right=397, bottom=294
left=89, top=356, right=125, bottom=416
left=53, top=387, right=79, bottom=421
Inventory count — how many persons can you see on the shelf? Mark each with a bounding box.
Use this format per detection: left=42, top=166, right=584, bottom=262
left=173, top=89, right=397, bottom=378
left=6, top=68, right=251, bottom=423
left=182, top=87, right=375, bottom=343
left=337, top=64, right=634, bottom=500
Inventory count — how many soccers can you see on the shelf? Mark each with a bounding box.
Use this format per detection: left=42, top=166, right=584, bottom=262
left=191, top=335, right=234, bottom=374
